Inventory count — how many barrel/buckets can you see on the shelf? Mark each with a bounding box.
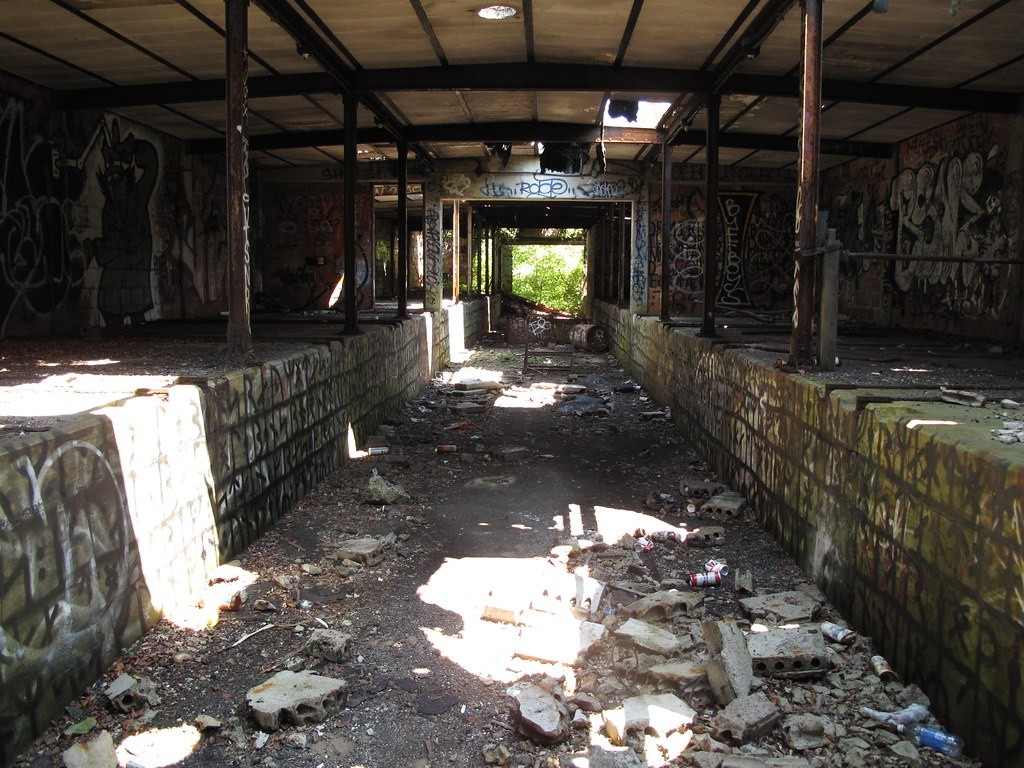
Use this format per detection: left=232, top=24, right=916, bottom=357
left=569, top=324, right=609, bottom=352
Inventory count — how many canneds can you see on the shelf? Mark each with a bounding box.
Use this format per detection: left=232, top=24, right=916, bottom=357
left=633, top=530, right=677, bottom=552
left=691, top=570, right=722, bottom=587
left=632, top=385, right=671, bottom=420
left=434, top=444, right=458, bottom=453
left=870, top=655, right=897, bottom=682
left=652, top=492, right=705, bottom=513
left=701, top=557, right=729, bottom=576
left=368, top=447, right=389, bottom=455
left=820, top=621, right=857, bottom=645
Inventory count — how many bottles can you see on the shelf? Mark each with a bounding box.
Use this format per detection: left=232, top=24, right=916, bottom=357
left=897, top=722, right=965, bottom=760
